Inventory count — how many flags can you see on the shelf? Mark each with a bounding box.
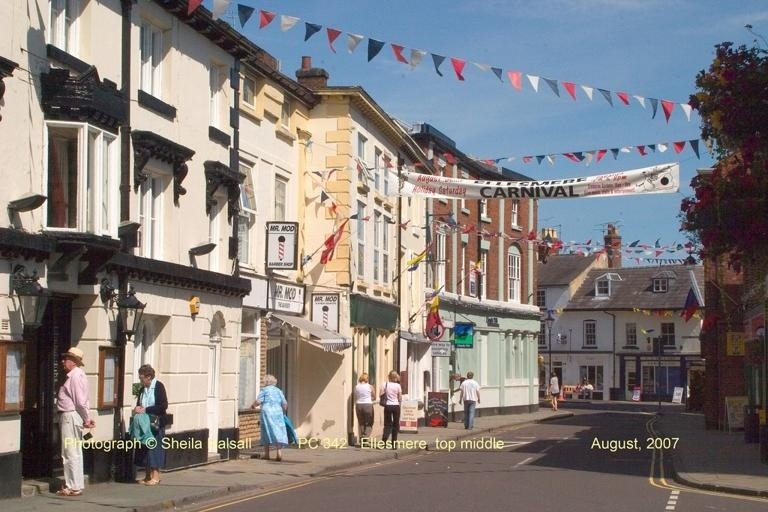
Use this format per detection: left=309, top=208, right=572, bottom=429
left=406, top=241, right=433, bottom=272
left=321, top=224, right=351, bottom=266
left=424, top=293, right=444, bottom=333
left=472, top=258, right=486, bottom=278
left=679, top=287, right=700, bottom=322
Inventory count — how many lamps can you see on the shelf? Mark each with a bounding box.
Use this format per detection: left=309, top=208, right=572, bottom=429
left=8, top=263, right=49, bottom=334
left=117, top=219, right=142, bottom=240
left=7, top=193, right=48, bottom=213
left=187, top=239, right=217, bottom=258
left=100, top=275, right=148, bottom=342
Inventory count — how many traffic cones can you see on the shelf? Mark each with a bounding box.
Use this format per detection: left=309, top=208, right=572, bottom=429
left=557, top=385, right=565, bottom=401
left=543, top=386, right=549, bottom=399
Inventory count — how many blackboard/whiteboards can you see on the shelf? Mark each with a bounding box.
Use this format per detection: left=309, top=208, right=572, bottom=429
left=428, top=392, right=448, bottom=425
left=726, top=396, right=750, bottom=428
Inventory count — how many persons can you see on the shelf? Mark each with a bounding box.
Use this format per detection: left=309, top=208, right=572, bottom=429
left=460, top=370, right=481, bottom=430
left=131, top=364, right=168, bottom=486
left=380, top=370, right=403, bottom=448
left=354, top=373, right=375, bottom=444
left=452, top=375, right=466, bottom=393
left=56, top=346, right=95, bottom=496
left=581, top=381, right=594, bottom=399
left=549, top=372, right=559, bottom=413
left=251, top=374, right=288, bottom=460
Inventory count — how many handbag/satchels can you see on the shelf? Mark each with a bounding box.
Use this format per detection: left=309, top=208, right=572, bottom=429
left=380, top=393, right=390, bottom=406
left=150, top=414, right=161, bottom=433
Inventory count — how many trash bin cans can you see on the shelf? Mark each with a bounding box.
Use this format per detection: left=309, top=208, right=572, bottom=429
left=742, top=405, right=762, bottom=443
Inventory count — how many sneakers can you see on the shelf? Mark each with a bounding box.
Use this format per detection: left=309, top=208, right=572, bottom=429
left=57, top=488, right=82, bottom=496
left=137, top=477, right=160, bottom=486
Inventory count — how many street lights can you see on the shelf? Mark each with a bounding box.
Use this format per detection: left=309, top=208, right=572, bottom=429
left=545, top=312, right=556, bottom=387
left=598, top=309, right=616, bottom=403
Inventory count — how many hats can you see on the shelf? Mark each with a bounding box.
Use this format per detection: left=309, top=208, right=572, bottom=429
left=62, top=348, right=84, bottom=367
